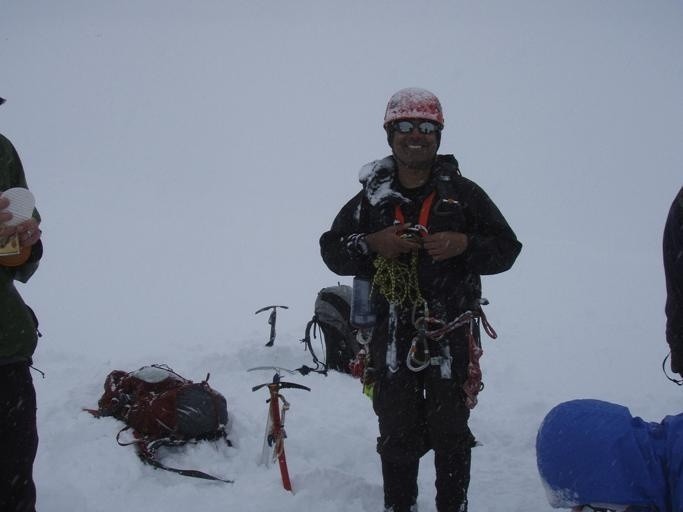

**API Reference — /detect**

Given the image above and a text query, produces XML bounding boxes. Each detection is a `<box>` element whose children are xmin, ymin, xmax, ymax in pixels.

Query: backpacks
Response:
<box><xmin>98</xmin><ymin>364</ymin><xmax>228</xmax><ymax>447</ymax></box>
<box><xmin>299</xmin><ymin>285</ymin><xmax>362</xmax><ymax>378</ymax></box>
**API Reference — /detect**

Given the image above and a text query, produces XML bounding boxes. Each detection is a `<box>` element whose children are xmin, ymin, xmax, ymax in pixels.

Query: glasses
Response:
<box><xmin>391</xmin><ymin>120</ymin><xmax>441</xmax><ymax>134</ymax></box>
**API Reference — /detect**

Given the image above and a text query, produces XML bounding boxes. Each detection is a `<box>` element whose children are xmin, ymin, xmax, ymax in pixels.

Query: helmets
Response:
<box><xmin>383</xmin><ymin>87</ymin><xmax>444</xmax><ymax>132</ymax></box>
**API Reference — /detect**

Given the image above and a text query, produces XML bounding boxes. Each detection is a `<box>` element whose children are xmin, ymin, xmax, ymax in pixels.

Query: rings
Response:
<box><xmin>27</xmin><ymin>230</ymin><xmax>33</xmax><ymax>235</ymax></box>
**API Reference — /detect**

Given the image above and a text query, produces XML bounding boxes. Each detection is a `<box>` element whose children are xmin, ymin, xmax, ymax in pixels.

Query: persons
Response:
<box><xmin>0</xmin><ymin>92</ymin><xmax>45</xmax><ymax>509</ymax></box>
<box><xmin>661</xmin><ymin>184</ymin><xmax>683</xmax><ymax>391</ymax></box>
<box><xmin>319</xmin><ymin>88</ymin><xmax>523</xmax><ymax>512</ymax></box>
<box><xmin>536</xmin><ymin>400</ymin><xmax>683</xmax><ymax>511</ymax></box>
